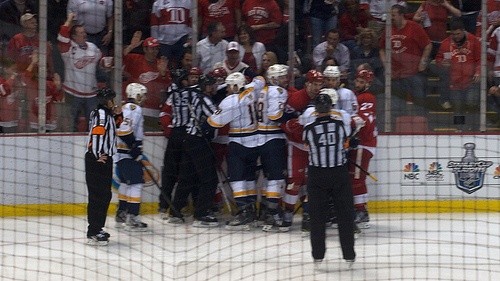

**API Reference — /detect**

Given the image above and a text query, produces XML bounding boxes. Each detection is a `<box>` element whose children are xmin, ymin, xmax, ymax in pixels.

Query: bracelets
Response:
<box><xmin>109</xmin><ymin>30</ymin><xmax>112</xmax><ymax>32</ymax></box>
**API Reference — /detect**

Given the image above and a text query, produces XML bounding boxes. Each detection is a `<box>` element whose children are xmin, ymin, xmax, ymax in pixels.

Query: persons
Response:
<box><xmin>115</xmin><ymin>83</ymin><xmax>149</xmax><ymax>233</ymax></box>
<box><xmin>85</xmin><ymin>89</ymin><xmax>122</xmax><ymax>246</ymax></box>
<box><xmin>0</xmin><ymin>0</ymin><xmax>500</xmax><ymax>132</ymax></box>
<box><xmin>302</xmin><ymin>95</ymin><xmax>355</xmax><ymax>264</ymax></box>
<box><xmin>159</xmin><ymin>65</ymin><xmax>376</xmax><ymax>233</ymax></box>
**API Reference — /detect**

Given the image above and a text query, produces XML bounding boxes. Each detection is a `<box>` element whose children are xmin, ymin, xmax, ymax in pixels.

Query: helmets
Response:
<box><xmin>125</xmin><ymin>83</ymin><xmax>147</xmax><ymax>103</ymax></box>
<box><xmin>319</xmin><ymin>87</ymin><xmax>338</xmax><ymax>107</ymax></box>
<box><xmin>188</xmin><ymin>67</ymin><xmax>203</xmax><ymax>76</ymax></box>
<box><xmin>225</xmin><ymin>72</ymin><xmax>246</xmax><ymax>93</ymax></box>
<box><xmin>324</xmin><ymin>66</ymin><xmax>341</xmax><ymax>88</ymax></box>
<box><xmin>210</xmin><ymin>67</ymin><xmax>226</xmax><ymax>79</ymax></box>
<box><xmin>305</xmin><ymin>69</ymin><xmax>323</xmax><ymax>92</ymax></box>
<box><xmin>95</xmin><ymin>87</ymin><xmax>117</xmax><ymax>107</ymax></box>
<box><xmin>356</xmin><ymin>69</ymin><xmax>374</xmax><ymax>92</ymax></box>
<box><xmin>265</xmin><ymin>63</ymin><xmax>288</xmax><ymax>86</ymax></box>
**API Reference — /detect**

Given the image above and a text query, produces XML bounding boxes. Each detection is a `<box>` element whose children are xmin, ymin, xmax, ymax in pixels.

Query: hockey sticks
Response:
<box><xmin>141</xmin><ymin>161</ymin><xmax>186</xmax><ymax>224</ymax></box>
<box><xmin>186</xmin><ymin>101</ymin><xmax>247</xmax><ymax>217</ymax></box>
<box><xmin>345</xmin><ymin>156</ymin><xmax>378</xmax><ymax>182</ymax></box>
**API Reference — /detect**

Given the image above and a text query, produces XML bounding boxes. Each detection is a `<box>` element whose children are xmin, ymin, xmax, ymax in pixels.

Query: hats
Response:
<box><xmin>142</xmin><ymin>37</ymin><xmax>161</xmax><ymax>49</ymax></box>
<box><xmin>226</xmin><ymin>41</ymin><xmax>240</xmax><ymax>51</ymax></box>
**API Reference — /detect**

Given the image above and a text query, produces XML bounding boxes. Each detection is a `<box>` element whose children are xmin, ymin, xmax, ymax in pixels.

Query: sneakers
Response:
<box><xmin>354</xmin><ymin>210</ymin><xmax>371</xmax><ymax>239</ymax></box>
<box><xmin>86</xmin><ymin>229</ymin><xmax>110</xmax><ymax>246</ymax></box>
<box><xmin>301</xmin><ymin>215</ymin><xmax>310</xmax><ymax>237</ymax></box>
<box><xmin>154</xmin><ymin>210</ymin><xmax>294</xmax><ymax>232</ymax></box>
<box><xmin>346</xmin><ymin>259</ymin><xmax>356</xmax><ymax>268</ymax></box>
<box><xmin>115</xmin><ymin>210</ymin><xmax>149</xmax><ymax>231</ymax></box>
<box><xmin>313</xmin><ymin>258</ymin><xmax>323</xmax><ymax>269</ymax></box>
